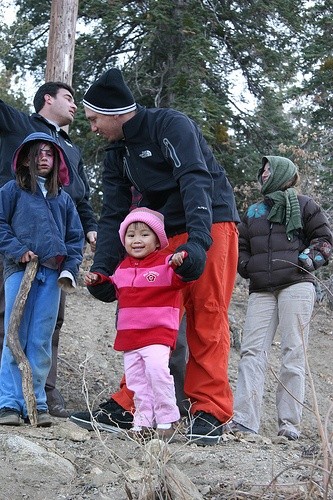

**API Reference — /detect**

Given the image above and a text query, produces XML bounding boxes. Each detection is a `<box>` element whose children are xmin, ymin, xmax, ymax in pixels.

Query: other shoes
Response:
<box><xmin>23</xmin><ymin>409</ymin><xmax>52</xmax><ymax>427</ymax></box>
<box><xmin>220</xmin><ymin>420</ymin><xmax>253</xmax><ymax>435</ymax></box>
<box><xmin>69</xmin><ymin>398</ymin><xmax>132</xmax><ymax>433</ymax></box>
<box><xmin>115</xmin><ymin>425</ymin><xmax>152</xmax><ymax>440</ymax></box>
<box><xmin>185</xmin><ymin>411</ymin><xmax>221</xmax><ymax>447</ymax></box>
<box><xmin>276</xmin><ymin>430</ymin><xmax>298</xmax><ymax>441</ymax></box>
<box><xmin>48</xmin><ymin>404</ymin><xmax>74</xmax><ymax>418</ymax></box>
<box><xmin>153</xmin><ymin>422</ymin><xmax>175</xmax><ymax>442</ymax></box>
<box><xmin>0</xmin><ymin>408</ymin><xmax>20</xmax><ymax>426</ymax></box>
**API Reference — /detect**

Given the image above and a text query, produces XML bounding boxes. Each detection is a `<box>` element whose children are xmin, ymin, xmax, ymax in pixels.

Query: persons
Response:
<box><xmin>222</xmin><ymin>157</ymin><xmax>333</xmax><ymax>439</ymax></box>
<box><xmin>0</xmin><ymin>132</ymin><xmax>83</xmax><ymax>426</ymax></box>
<box><xmin>0</xmin><ymin>81</ymin><xmax>97</xmax><ymax>417</ymax></box>
<box><xmin>84</xmin><ymin>207</ymin><xmax>188</xmax><ymax>442</ymax></box>
<box><xmin>70</xmin><ymin>69</ymin><xmax>237</xmax><ymax>443</ymax></box>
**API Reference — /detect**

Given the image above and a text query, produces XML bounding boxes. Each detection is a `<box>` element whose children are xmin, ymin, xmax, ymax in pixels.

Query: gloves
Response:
<box><xmin>86</xmin><ymin>262</ymin><xmax>115</xmax><ymax>303</ymax></box>
<box><xmin>170</xmin><ymin>230</ymin><xmax>213</xmax><ymax>282</ymax></box>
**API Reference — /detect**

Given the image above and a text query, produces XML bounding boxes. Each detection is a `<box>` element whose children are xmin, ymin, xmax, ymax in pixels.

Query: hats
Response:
<box><xmin>119</xmin><ymin>207</ymin><xmax>168</xmax><ymax>250</ymax></box>
<box><xmin>82</xmin><ymin>68</ymin><xmax>135</xmax><ymax>115</ymax></box>
